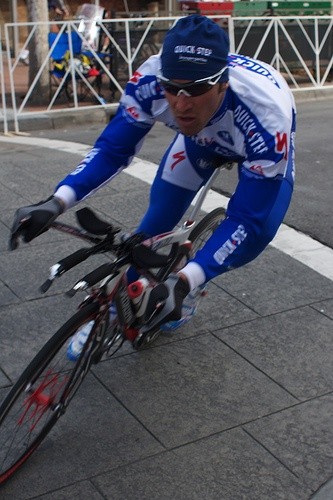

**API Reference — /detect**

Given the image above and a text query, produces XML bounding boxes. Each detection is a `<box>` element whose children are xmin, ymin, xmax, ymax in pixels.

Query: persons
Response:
<box><xmin>9</xmin><ymin>15</ymin><xmax>299</xmax><ymax>360</ymax></box>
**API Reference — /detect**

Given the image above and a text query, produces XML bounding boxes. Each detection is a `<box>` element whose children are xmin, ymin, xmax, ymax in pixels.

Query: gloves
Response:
<box><xmin>9</xmin><ymin>195</ymin><xmax>64</xmax><ymax>251</ymax></box>
<box><xmin>137</xmin><ymin>273</ymin><xmax>190</xmax><ymax>330</ymax></box>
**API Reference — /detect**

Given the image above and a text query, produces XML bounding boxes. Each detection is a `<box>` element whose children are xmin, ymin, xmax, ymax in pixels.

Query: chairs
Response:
<box><xmin>0</xmin><ymin>0</ymin><xmax>113</xmax><ymax>104</ymax></box>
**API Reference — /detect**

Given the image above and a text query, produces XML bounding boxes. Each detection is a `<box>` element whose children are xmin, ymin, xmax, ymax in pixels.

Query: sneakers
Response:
<box><xmin>67</xmin><ymin>320</ymin><xmax>95</xmax><ymax>361</ymax></box>
<box><xmin>160</xmin><ymin>283</ymin><xmax>207</xmax><ymax>333</ymax></box>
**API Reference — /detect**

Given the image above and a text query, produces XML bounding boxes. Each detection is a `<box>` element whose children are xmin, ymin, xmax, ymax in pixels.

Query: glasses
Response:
<box><xmin>158</xmin><ymin>64</ymin><xmax>227</xmax><ymax>97</ymax></box>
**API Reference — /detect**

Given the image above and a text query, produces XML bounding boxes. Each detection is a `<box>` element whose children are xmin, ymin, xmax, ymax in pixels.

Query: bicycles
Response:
<box><xmin>0</xmin><ymin>167</ymin><xmax>228</xmax><ymax>483</ymax></box>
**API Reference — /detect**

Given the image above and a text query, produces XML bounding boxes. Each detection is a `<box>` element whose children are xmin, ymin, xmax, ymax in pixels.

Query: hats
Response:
<box><xmin>161</xmin><ymin>15</ymin><xmax>230</xmax><ymax>82</ymax></box>
